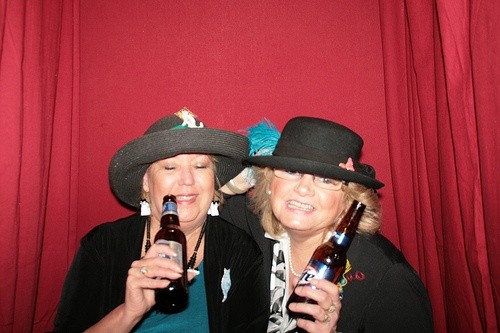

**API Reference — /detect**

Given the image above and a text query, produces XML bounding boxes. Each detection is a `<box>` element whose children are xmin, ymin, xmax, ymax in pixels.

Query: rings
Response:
<box><xmin>324</xmin><ymin>306</ymin><xmax>335</xmax><ymax>313</ymax></box>
<box><xmin>317</xmin><ymin>315</ymin><xmax>330</xmax><ymax>322</ymax></box>
<box><xmin>139</xmin><ymin>270</ymin><xmax>148</xmax><ymax>275</ymax></box>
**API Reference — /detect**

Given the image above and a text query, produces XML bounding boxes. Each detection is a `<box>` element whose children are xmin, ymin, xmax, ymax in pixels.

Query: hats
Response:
<box><xmin>108</xmin><ymin>111</ymin><xmax>251</xmax><ymax>212</ymax></box>
<box><xmin>246</xmin><ymin>116</ymin><xmax>385</xmax><ymax>191</ymax></box>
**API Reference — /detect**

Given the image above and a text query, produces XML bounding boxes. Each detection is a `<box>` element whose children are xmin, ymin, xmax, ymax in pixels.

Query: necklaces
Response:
<box><xmin>146</xmin><ymin>216</ymin><xmax>208</xmax><ymax>282</ymax></box>
<box><xmin>288</xmin><ymin>238</ymin><xmax>305</xmax><ymax>278</ymax></box>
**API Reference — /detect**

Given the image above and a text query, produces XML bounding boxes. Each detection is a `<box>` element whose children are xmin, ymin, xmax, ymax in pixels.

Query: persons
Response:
<box><xmin>54</xmin><ymin>111</ymin><xmax>274</xmax><ymax>333</ymax></box>
<box><xmin>241</xmin><ymin>117</ymin><xmax>437</xmax><ymax>333</ymax></box>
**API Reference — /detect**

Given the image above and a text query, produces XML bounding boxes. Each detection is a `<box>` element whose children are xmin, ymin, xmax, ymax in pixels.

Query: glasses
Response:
<box><xmin>273</xmin><ymin>166</ymin><xmax>348</xmax><ymax>191</ymax></box>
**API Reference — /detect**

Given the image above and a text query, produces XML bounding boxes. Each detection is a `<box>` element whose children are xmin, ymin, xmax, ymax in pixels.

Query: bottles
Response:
<box><xmin>154</xmin><ymin>194</ymin><xmax>189</xmax><ymax>315</ymax></box>
<box><xmin>286</xmin><ymin>200</ymin><xmax>366</xmax><ymax>322</ymax></box>
